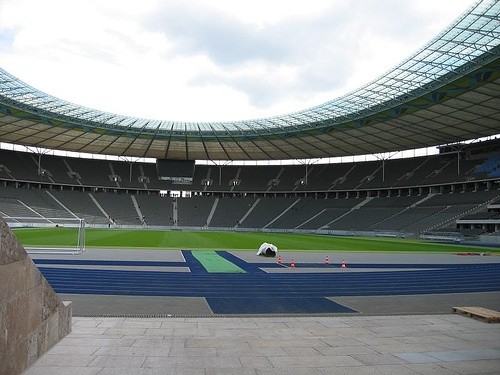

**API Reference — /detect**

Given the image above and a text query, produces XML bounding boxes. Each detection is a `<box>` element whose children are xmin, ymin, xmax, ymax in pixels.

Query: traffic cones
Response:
<box><xmin>340</xmin><ymin>259</ymin><xmax>347</xmax><ymax>269</ymax></box>
<box><xmin>278</xmin><ymin>255</ymin><xmax>283</xmax><ymax>264</ymax></box>
<box><xmin>325</xmin><ymin>256</ymin><xmax>331</xmax><ymax>265</ymax></box>
<box><xmin>288</xmin><ymin>259</ymin><xmax>296</xmax><ymax>268</ymax></box>
